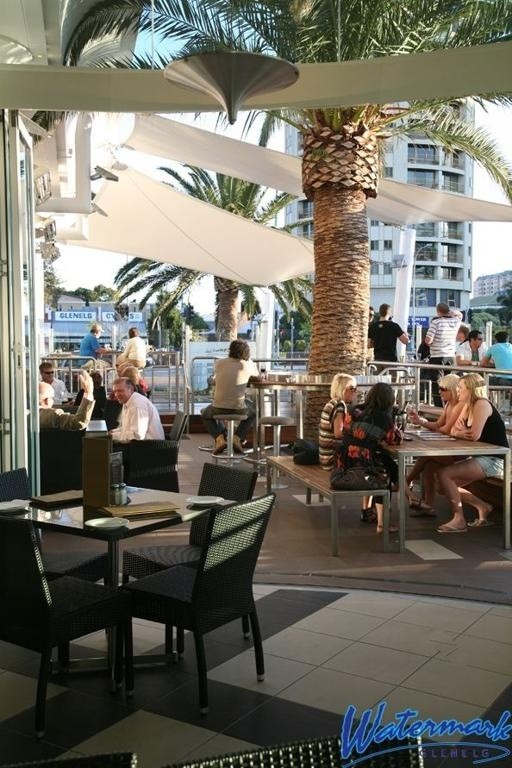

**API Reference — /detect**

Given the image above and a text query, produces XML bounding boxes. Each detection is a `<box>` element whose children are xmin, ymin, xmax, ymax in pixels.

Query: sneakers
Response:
<box><xmin>359</xmin><ymin>507</ymin><xmax>379</xmax><ymax>524</ymax></box>
<box><xmin>146</xmin><ymin>389</ymin><xmax>153</xmax><ymax>400</ymax></box>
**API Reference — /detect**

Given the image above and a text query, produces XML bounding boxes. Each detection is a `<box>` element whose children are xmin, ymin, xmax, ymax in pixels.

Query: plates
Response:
<box><xmin>186</xmin><ymin>503</ymin><xmax>223</xmax><ymax>510</ymax></box>
<box><xmin>186</xmin><ymin>496</ymin><xmax>224</xmax><ymax>505</ymax></box>
<box><xmin>83</xmin><ymin>517</ymin><xmax>131</xmax><ymax>530</ymax></box>
<box><xmin>1</xmin><ymin>499</ymin><xmax>30</xmax><ymax>513</ymax></box>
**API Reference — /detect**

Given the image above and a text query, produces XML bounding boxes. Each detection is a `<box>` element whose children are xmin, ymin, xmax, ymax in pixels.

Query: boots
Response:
<box><xmin>213</xmin><ymin>434</ymin><xmax>228</xmax><ymax>455</ymax></box>
<box><xmin>226</xmin><ymin>434</ymin><xmax>245</xmax><ymax>453</ymax></box>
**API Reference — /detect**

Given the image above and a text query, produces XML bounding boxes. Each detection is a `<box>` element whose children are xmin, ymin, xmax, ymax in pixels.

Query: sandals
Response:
<box><xmin>408</xmin><ymin>497</ymin><xmax>439</xmax><ymax>517</ymax></box>
<box><xmin>376</xmin><ymin>523</ymin><xmax>400</xmax><ymax>534</ymax></box>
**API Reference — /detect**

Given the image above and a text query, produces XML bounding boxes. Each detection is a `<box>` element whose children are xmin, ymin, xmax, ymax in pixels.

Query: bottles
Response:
<box><xmin>110</xmin><ymin>483</ymin><xmax>128</xmax><ymax>506</ymax></box>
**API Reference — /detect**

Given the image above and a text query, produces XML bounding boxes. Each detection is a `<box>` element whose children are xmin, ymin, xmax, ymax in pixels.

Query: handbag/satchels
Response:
<box><xmin>285</xmin><ymin>438</ymin><xmax>318</xmax><ymax>465</ymax></box>
<box><xmin>329</xmin><ymin>463</ymin><xmax>387</xmax><ymax>490</ymax></box>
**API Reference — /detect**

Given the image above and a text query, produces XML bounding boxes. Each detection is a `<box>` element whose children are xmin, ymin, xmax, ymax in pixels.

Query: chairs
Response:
<box><xmin>1</xmin><ymin>352</ymin><xmax>512</xmax><ymax>767</ymax></box>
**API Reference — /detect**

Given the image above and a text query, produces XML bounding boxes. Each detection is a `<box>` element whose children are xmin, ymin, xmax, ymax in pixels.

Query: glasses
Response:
<box><xmin>347</xmin><ymin>388</ymin><xmax>357</xmax><ymax>392</ymax></box>
<box><xmin>42</xmin><ymin>371</ymin><xmax>54</xmax><ymax>374</ymax></box>
<box><xmin>439</xmin><ymin>387</ymin><xmax>447</xmax><ymax>391</ymax></box>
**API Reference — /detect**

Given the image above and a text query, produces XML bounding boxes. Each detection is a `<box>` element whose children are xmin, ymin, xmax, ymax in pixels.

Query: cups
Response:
<box><xmin>399</xmin><ymin>352</ymin><xmax>421</xmax><ymax>362</ymax></box>
<box><xmin>354</xmin><ymin>375</ymin><xmax>391</xmax><ymax>384</ymax></box>
<box><xmin>395</xmin><ymin>414</ymin><xmax>407</xmax><ymax>436</ymax></box>
<box><xmin>290</xmin><ymin>371</ymin><xmax>327</xmax><ymax>383</ymax></box>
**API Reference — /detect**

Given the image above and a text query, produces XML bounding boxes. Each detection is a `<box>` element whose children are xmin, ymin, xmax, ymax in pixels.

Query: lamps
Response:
<box><xmin>89</xmin><ymin>164</ymin><xmax>119</xmax><ymax>183</ymax></box>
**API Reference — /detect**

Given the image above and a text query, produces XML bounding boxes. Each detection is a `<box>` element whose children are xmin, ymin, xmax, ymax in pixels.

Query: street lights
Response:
<box><xmin>412</xmin><ymin>241</ymin><xmax>435</xmax><ymax>360</ymax></box>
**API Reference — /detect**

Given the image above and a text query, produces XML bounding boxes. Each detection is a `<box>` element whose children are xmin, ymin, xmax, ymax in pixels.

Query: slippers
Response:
<box><xmin>434</xmin><ymin>524</ymin><xmax>468</xmax><ymax>533</ymax></box>
<box><xmin>467</xmin><ymin>518</ymin><xmax>496</xmax><ymax>527</ymax></box>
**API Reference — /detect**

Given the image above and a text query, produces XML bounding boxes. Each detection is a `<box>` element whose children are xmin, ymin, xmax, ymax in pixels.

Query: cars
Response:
<box><xmin>404</xmin><ymin>351</ymin><xmax>429</xmax><ymax>365</ymax></box>
<box><xmin>60</xmin><ymin>350</ymin><xmax>85</xmax><ymax>383</ymax></box>
<box><xmin>146</xmin><ymin>354</ymin><xmax>155</xmax><ymax>369</ymax></box>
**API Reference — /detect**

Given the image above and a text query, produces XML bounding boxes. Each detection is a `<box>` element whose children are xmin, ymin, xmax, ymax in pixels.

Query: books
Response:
<box><xmin>102</xmin><ymin>499</ymin><xmax>180</xmax><ymax>517</ymax></box>
<box><xmin>28</xmin><ymin>488</ymin><xmax>84</xmax><ymax>510</ymax></box>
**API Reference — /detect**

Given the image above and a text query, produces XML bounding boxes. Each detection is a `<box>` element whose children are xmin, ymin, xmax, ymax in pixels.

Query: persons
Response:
<box><xmin>110</xmin><ymin>376</ymin><xmax>166</xmax><ymax>443</ymax></box>
<box><xmin>368</xmin><ymin>302</ymin><xmax>512</xmax><ymax>407</ymax></box>
<box><xmin>35</xmin><ymin>322</ymin><xmax>148</xmax><ymax>430</ymax></box>
<box><xmin>436</xmin><ymin>371</ymin><xmax>509</xmax><ymax>532</ymax></box>
<box><xmin>334</xmin><ymin>382</ymin><xmax>437</xmax><ymax>532</ymax></box>
<box><xmin>201</xmin><ymin>340</ymin><xmax>260</xmax><ymax>454</ymax></box>
<box><xmin>317</xmin><ymin>373</ymin><xmax>386</xmax><ymax>524</ymax></box>
<box><xmin>405</xmin><ymin>372</ymin><xmax>470</xmax><ymax>518</ymax></box>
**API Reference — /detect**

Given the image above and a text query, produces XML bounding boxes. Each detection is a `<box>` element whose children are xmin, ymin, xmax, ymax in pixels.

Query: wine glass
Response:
<box><xmin>260</xmin><ymin>364</ymin><xmax>266</xmax><ymax>382</ymax></box>
<box><xmin>406</xmin><ymin>404</ymin><xmax>416</xmax><ymax>428</ymax></box>
